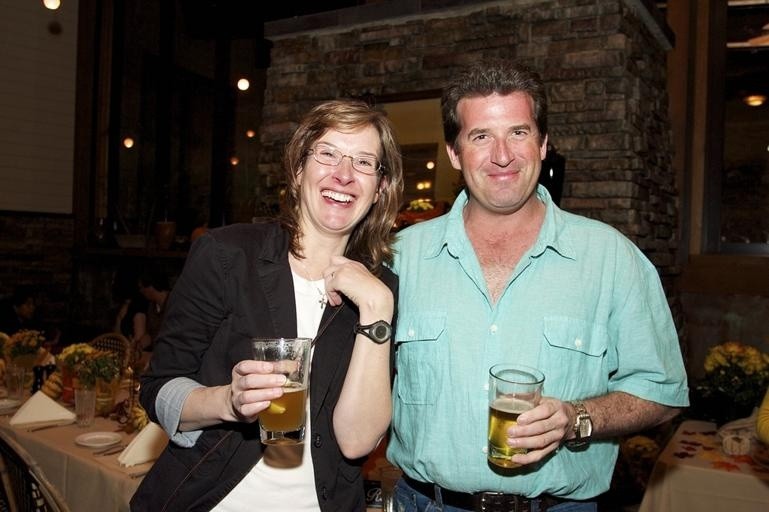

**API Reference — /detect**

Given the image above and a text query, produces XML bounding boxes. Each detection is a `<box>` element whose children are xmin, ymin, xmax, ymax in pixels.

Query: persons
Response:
<box><xmin>0</xmin><ymin>284</ymin><xmax>54</xmax><ymax>343</ymax></box>
<box><xmin>379</xmin><ymin>54</ymin><xmax>696</xmax><ymax>511</ymax></box>
<box><xmin>101</xmin><ymin>269</ymin><xmax>147</xmax><ymax>346</ymax></box>
<box><xmin>137</xmin><ymin>265</ymin><xmax>171</xmax><ymax>353</ymax></box>
<box><xmin>127</xmin><ymin>96</ymin><xmax>401</xmax><ymax>511</ymax></box>
<box><xmin>756</xmin><ymin>385</ymin><xmax>769</xmax><ymax>449</ymax></box>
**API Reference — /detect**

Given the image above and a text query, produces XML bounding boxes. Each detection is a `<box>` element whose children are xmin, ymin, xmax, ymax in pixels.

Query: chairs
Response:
<box><xmin>1</xmin><ymin>431</ymin><xmax>69</xmax><ymax>512</ymax></box>
<box><xmin>86</xmin><ymin>331</ymin><xmax>134</xmax><ymax>416</ymax></box>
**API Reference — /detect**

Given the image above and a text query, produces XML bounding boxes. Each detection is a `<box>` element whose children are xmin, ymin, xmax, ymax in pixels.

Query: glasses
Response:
<box><xmin>305</xmin><ymin>142</ymin><xmax>388</xmax><ymax>175</ymax></box>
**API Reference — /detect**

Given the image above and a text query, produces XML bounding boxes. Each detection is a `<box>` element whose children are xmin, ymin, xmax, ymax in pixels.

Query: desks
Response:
<box><xmin>635</xmin><ymin>418</ymin><xmax>768</xmax><ymax>511</ymax></box>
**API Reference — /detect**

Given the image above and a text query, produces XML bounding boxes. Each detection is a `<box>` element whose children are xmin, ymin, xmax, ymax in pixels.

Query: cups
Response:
<box><xmin>250</xmin><ymin>336</ymin><xmax>311</xmax><ymax>444</ymax></box>
<box><xmin>484</xmin><ymin>363</ymin><xmax>546</xmax><ymax>468</ymax></box>
<box><xmin>6</xmin><ymin>366</ymin><xmax>25</xmax><ymax>403</ymax></box>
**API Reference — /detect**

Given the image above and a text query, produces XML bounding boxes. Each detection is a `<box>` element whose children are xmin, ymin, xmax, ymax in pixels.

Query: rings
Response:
<box><xmin>331</xmin><ymin>271</ymin><xmax>335</xmax><ymax>278</ymax></box>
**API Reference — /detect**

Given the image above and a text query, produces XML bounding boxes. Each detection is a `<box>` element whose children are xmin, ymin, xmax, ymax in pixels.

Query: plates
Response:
<box><xmin>0</xmin><ymin>398</ymin><xmax>22</xmax><ymax>410</ymax></box>
<box><xmin>75</xmin><ymin>431</ymin><xmax>122</xmax><ymax>447</ymax></box>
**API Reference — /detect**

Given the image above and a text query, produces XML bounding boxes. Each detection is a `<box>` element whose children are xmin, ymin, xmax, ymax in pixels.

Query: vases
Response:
<box><xmin>5</xmin><ymin>358</ymin><xmax>27</xmax><ymax>401</ymax></box>
<box><xmin>714</xmin><ymin>397</ymin><xmax>754</xmax><ymax>429</ymax></box>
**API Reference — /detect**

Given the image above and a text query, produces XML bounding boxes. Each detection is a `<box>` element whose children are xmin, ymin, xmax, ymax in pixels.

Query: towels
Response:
<box><xmin>10</xmin><ymin>387</ymin><xmax>80</xmax><ymax>430</ymax></box>
<box><xmin>118</xmin><ymin>419</ymin><xmax>170</xmax><ymax>466</ymax></box>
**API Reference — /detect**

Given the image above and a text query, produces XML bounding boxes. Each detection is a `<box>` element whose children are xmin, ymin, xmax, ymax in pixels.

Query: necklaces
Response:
<box><xmin>297</xmin><ymin>247</ymin><xmax>329</xmax><ymax>311</ymax></box>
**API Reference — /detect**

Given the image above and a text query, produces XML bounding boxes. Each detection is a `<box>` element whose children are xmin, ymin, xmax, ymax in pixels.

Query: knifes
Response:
<box><xmin>30</xmin><ymin>421</ymin><xmax>76</xmax><ymax>431</ymax></box>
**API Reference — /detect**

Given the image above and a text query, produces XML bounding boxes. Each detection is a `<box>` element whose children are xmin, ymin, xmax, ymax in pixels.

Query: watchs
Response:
<box><xmin>566</xmin><ymin>400</ymin><xmax>595</xmax><ymax>453</ymax></box>
<box><xmin>352</xmin><ymin>320</ymin><xmax>395</xmax><ymax>344</ymax></box>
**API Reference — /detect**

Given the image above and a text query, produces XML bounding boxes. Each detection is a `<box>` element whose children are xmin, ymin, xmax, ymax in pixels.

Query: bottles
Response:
<box><xmin>31</xmin><ymin>363</ymin><xmax>59</xmax><ymax>394</ymax></box>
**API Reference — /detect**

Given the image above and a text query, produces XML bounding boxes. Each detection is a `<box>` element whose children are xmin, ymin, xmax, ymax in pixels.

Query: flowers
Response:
<box><xmin>3</xmin><ymin>326</ymin><xmax>49</xmax><ymax>362</ymax></box>
<box><xmin>623</xmin><ymin>435</ymin><xmax>660</xmax><ymax>461</ymax></box>
<box><xmin>59</xmin><ymin>342</ymin><xmax>122</xmax><ymax>387</ymax></box>
<box><xmin>701</xmin><ymin>337</ymin><xmax>769</xmax><ymax>400</ymax></box>
